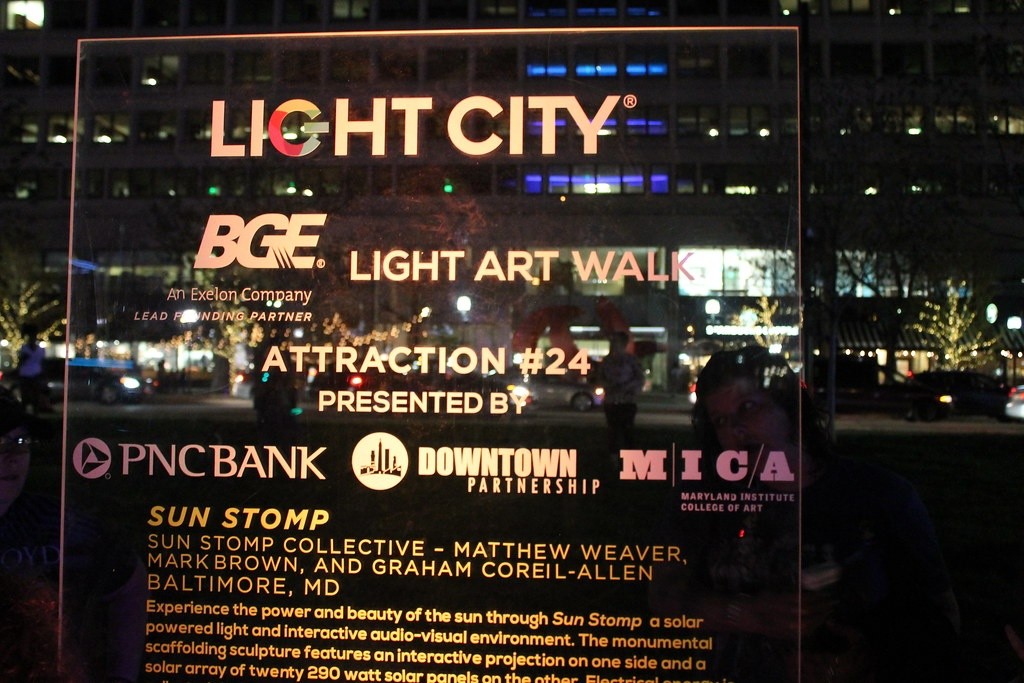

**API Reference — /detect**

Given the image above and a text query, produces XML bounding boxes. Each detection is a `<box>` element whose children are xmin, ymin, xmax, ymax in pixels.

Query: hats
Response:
<box><xmin>0</xmin><ymin>384</ymin><xmax>39</xmax><ymax>434</ymax></box>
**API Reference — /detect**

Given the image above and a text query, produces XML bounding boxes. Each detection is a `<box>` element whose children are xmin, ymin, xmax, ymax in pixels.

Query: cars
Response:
<box><xmin>14</xmin><ymin>313</ymin><xmax>891</xmax><ymax>419</ymax></box>
<box><xmin>1003</xmin><ymin>384</ymin><xmax>1024</xmax><ymax>422</ymax></box>
<box><xmin>884</xmin><ymin>368</ymin><xmax>1004</xmax><ymax>425</ymax></box>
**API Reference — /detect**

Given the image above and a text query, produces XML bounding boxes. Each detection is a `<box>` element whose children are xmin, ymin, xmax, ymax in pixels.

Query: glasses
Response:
<box><xmin>0</xmin><ymin>433</ymin><xmax>31</xmax><ymax>454</ymax></box>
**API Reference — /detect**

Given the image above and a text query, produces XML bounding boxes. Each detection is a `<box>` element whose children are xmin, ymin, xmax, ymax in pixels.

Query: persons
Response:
<box><xmin>18</xmin><ymin>338</ymin><xmax>47</xmax><ymax>412</ymax></box>
<box><xmin>586</xmin><ymin>330</ymin><xmax>648</xmax><ymax>453</ymax></box>
<box><xmin>251</xmin><ymin>337</ymin><xmax>297</xmax><ymax>456</ymax></box>
<box><xmin>650</xmin><ymin>347</ymin><xmax>962</xmax><ymax>683</ymax></box>
<box><xmin>0</xmin><ymin>384</ymin><xmax>146</xmax><ymax>682</ymax></box>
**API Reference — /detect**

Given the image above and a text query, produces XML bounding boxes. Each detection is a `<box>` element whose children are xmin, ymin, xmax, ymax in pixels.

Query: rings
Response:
<box><xmin>828</xmin><ymin>665</ymin><xmax>835</xmax><ymax>676</ymax></box>
<box><xmin>833</xmin><ymin>654</ymin><xmax>838</xmax><ymax>665</ymax></box>
